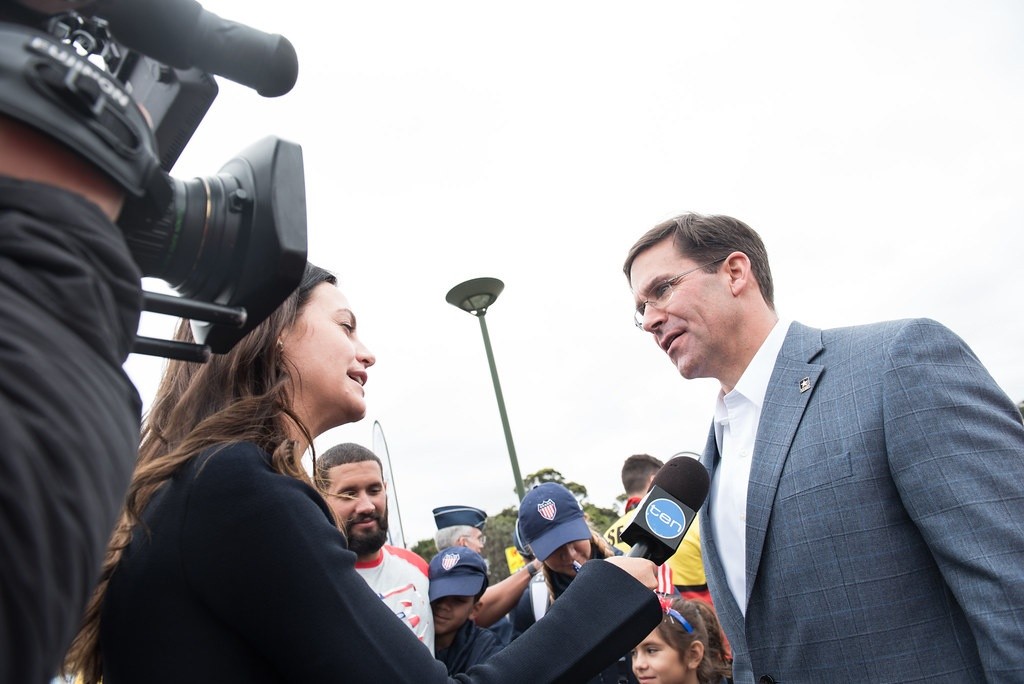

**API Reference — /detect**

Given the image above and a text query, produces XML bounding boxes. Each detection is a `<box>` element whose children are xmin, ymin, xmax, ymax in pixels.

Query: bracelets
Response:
<box><xmin>528</xmin><ymin>563</ymin><xmax>537</xmax><ymax>577</ymax></box>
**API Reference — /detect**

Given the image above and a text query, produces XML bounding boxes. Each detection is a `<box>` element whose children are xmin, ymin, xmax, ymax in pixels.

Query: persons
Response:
<box><xmin>433</xmin><ymin>505</ymin><xmax>543</xmax><ymax>647</ymax></box>
<box><xmin>631</xmin><ymin>598</ymin><xmax>732</xmax><ymax>684</ymax></box>
<box><xmin>511</xmin><ymin>483</ymin><xmax>682</xmax><ymax>648</ymax></box>
<box><xmin>624</xmin><ymin>213</ymin><xmax>1024</xmax><ymax>684</ymax></box>
<box><xmin>315</xmin><ymin>443</ymin><xmax>434</xmax><ymax>660</ymax></box>
<box><xmin>514</xmin><ymin>515</ymin><xmax>624</xmax><ymax>634</ymax></box>
<box><xmin>0</xmin><ymin>121</ymin><xmax>143</xmax><ymax>684</ymax></box>
<box><xmin>608</xmin><ymin>455</ymin><xmax>713</xmax><ymax>609</ymax></box>
<box><xmin>69</xmin><ymin>261</ymin><xmax>657</xmax><ymax>684</ymax></box>
<box><xmin>428</xmin><ymin>546</ymin><xmax>505</xmax><ymax>676</ymax></box>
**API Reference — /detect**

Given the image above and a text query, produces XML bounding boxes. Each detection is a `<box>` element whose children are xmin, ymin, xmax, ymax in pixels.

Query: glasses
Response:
<box><xmin>632</xmin><ymin>257</ymin><xmax>727</xmax><ymax>331</ymax></box>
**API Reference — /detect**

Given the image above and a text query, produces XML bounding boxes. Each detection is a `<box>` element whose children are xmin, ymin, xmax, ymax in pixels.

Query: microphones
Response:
<box><xmin>621</xmin><ymin>457</ymin><xmax>711</xmax><ymax>566</ymax></box>
<box><xmin>73</xmin><ymin>0</ymin><xmax>298</xmax><ymax>98</ymax></box>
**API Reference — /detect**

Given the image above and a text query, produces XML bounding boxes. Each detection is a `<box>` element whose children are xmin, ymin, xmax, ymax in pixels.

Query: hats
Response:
<box><xmin>433</xmin><ymin>505</ymin><xmax>487</xmax><ymax>531</ymax></box>
<box><xmin>520</xmin><ymin>482</ymin><xmax>592</xmax><ymax>561</ymax></box>
<box><xmin>513</xmin><ymin>518</ymin><xmax>531</xmax><ymax>556</ymax></box>
<box><xmin>428</xmin><ymin>546</ymin><xmax>487</xmax><ymax>601</ymax></box>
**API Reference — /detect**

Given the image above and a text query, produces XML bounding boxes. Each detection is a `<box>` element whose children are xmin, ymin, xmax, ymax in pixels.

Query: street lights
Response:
<box><xmin>443</xmin><ymin>276</ymin><xmax>526</xmax><ymax>502</ymax></box>
